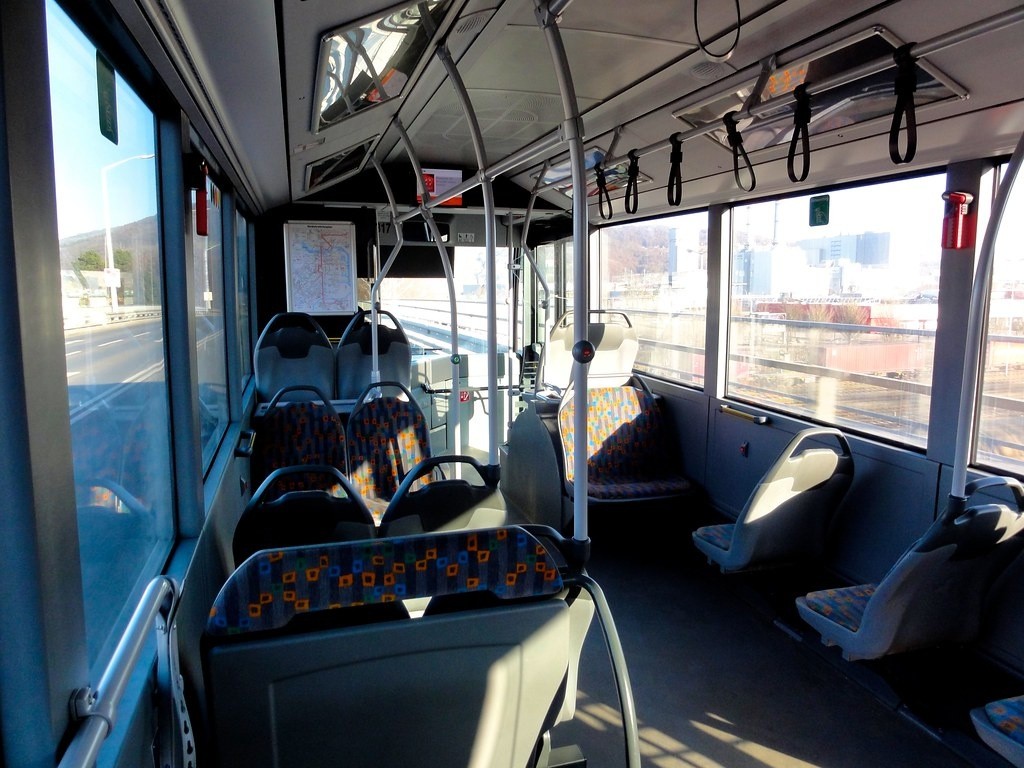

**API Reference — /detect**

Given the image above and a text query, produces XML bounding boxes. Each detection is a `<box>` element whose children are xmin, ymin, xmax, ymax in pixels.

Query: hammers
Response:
<box><xmin>941</xmin><ymin>192</ymin><xmax>973</xmax><ymax>214</ymax></box>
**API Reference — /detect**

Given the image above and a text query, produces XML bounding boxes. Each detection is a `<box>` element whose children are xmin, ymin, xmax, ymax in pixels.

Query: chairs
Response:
<box><xmin>197</xmin><ymin>310</ymin><xmax>599</xmax><ymax>768</ymax></box>
<box><xmin>691</xmin><ymin>427</ymin><xmax>856</xmax><ymax>572</ymax></box>
<box><xmin>558</xmin><ymin>374</ymin><xmax>689</xmax><ymax>528</ymax></box>
<box><xmin>794</xmin><ymin>475</ymin><xmax>1024</xmax><ymax>662</ymax></box>
<box><xmin>521</xmin><ymin>310</ymin><xmax>639</xmax><ymax>405</ymax></box>
<box><xmin>968</xmin><ymin>692</ymin><xmax>1024</xmax><ymax>768</ymax></box>
